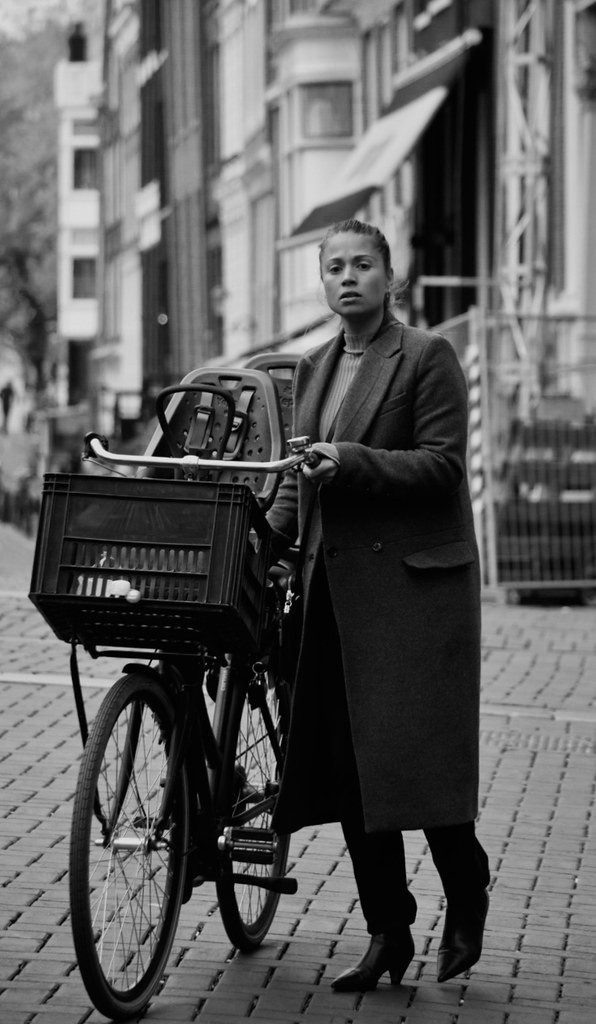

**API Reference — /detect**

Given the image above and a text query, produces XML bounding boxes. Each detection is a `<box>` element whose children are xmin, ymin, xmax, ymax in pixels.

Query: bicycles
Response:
<box><xmin>29</xmin><ymin>434</ymin><xmax>320</xmax><ymax>1024</ymax></box>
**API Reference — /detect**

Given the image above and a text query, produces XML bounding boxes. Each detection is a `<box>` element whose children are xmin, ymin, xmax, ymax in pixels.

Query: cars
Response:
<box><xmin>0</xmin><ymin>389</ymin><xmax>87</xmax><ymax>537</ymax></box>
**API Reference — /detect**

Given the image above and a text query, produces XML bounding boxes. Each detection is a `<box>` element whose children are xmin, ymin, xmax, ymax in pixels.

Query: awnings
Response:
<box><xmin>273</xmin><ymin>30</ymin><xmax>492</xmax><ymax>255</ymax></box>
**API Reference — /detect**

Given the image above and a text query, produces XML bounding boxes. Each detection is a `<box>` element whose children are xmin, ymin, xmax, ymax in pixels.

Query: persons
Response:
<box><xmin>67</xmin><ymin>19</ymin><xmax>88</xmax><ymax>62</ymax></box>
<box><xmin>0</xmin><ymin>381</ymin><xmax>15</xmax><ymax>429</ymax></box>
<box><xmin>245</xmin><ymin>220</ymin><xmax>491</xmax><ymax>991</ymax></box>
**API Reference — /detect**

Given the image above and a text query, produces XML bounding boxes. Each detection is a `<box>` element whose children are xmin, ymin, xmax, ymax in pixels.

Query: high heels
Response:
<box><xmin>330</xmin><ymin>918</ymin><xmax>416</xmax><ymax>993</ymax></box>
<box><xmin>437</xmin><ymin>890</ymin><xmax>490</xmax><ymax>983</ymax></box>
